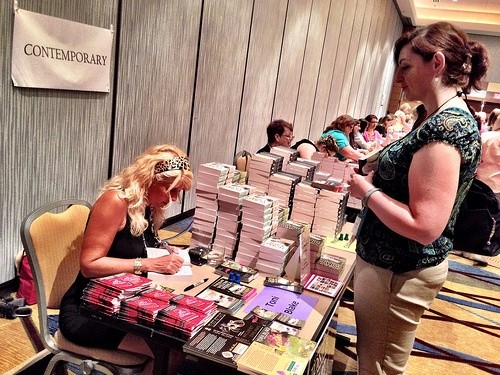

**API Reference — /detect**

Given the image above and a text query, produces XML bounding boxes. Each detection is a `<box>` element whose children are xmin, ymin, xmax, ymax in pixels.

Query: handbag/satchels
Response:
<box><xmin>453</xmin><ymin>105</ymin><xmax>500</xmax><ymax>257</ymax></box>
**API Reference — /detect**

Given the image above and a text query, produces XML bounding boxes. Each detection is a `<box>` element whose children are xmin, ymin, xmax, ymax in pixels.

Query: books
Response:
<box><xmin>81</xmin><ymin>248</ymin><xmax>318</xmax><ymax>375</ymax></box>
<box><xmin>190</xmin><ymin>145</ymin><xmax>365</xmax><ymax>298</ymax></box>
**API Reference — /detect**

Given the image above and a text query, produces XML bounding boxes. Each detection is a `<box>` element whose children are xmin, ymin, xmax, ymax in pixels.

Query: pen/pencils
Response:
<box><xmin>163</xmin><ymin>242</ymin><xmax>173</xmax><ymax>254</ymax></box>
<box><xmin>183</xmin><ymin>277</ymin><xmax>208</xmax><ymax>292</ymax></box>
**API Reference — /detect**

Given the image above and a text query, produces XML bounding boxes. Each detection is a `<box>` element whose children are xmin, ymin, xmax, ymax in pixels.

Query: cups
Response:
<box><xmin>207</xmin><ymin>245</ymin><xmax>225</xmax><ymax>267</ymax></box>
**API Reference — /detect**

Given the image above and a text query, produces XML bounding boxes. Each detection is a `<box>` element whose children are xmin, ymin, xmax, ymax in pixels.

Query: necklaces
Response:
<box><xmin>142</xmin><ymin>204</ymin><xmax>161</xmax><ymax>249</ymax></box>
<box><xmin>378</xmin><ymin>91</ymin><xmax>464</xmax><ymax>152</ymax></box>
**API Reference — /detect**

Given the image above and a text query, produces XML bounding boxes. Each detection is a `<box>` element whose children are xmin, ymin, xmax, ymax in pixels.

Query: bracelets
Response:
<box><xmin>133</xmin><ymin>258</ymin><xmax>142</xmax><ymax>273</ymax></box>
<box><xmin>362</xmin><ymin>188</ymin><xmax>382</xmax><ymax>208</ymax></box>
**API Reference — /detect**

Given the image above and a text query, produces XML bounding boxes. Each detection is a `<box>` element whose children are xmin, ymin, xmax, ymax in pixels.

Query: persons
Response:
<box><xmin>346</xmin><ymin>21</ymin><xmax>491</xmax><ymax>375</ymax></box>
<box><xmin>59</xmin><ymin>144</ymin><xmax>194</xmax><ymax>375</ymax></box>
<box><xmin>290</xmin><ymin>135</ymin><xmax>339</xmax><ymax>162</ymax></box>
<box><xmin>256</xmin><ymin>119</ymin><xmax>294</xmax><ymax>153</ymax></box>
<box><xmin>453</xmin><ymin>105</ymin><xmax>500</xmax><ymax>257</ymax></box>
<box><xmin>321</xmin><ymin>103</ymin><xmax>419</xmax><ymax>160</ymax></box>
